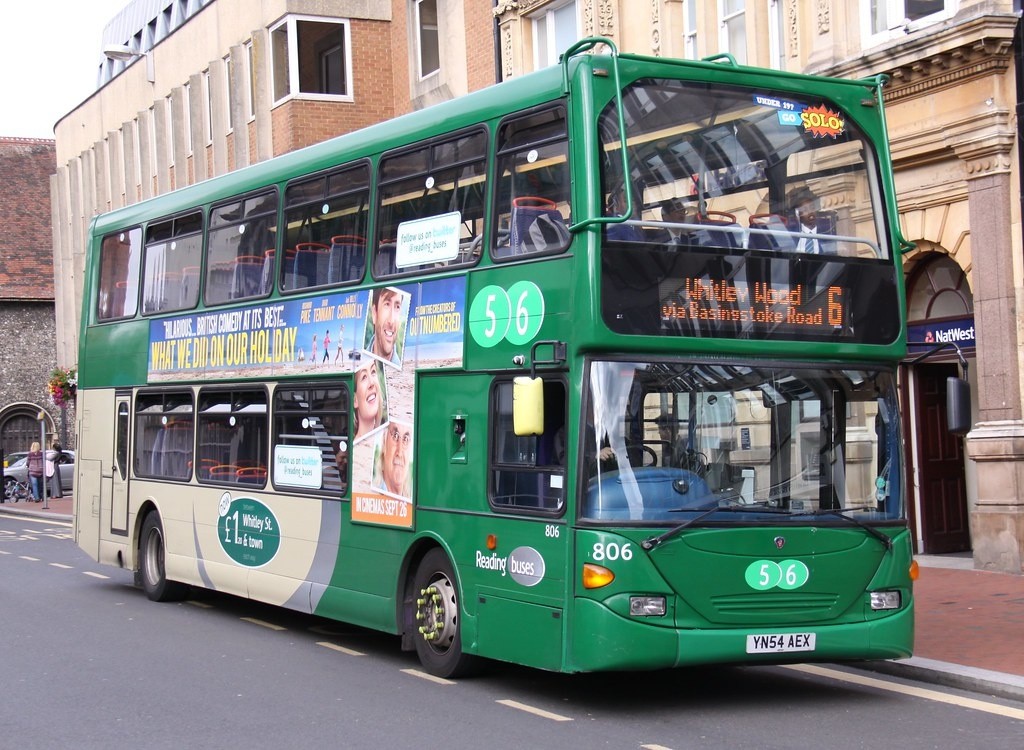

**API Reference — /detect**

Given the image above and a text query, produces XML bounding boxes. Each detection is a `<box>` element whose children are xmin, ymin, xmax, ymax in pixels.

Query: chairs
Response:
<box><xmin>150</xmin><ymin>420</ymin><xmax>267</xmax><ymax>486</ymax></box>
<box><xmin>605</xmin><ymin>211</ymin><xmax>797</xmax><ymax>253</ymax></box>
<box><xmin>104</xmin><ymin>196</ymin><xmax>569</xmax><ymax>318</ymax></box>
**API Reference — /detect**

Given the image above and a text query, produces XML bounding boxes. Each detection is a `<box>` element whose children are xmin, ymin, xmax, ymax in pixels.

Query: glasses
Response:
<box><xmin>389</xmin><ymin>429</ymin><xmax>411</xmax><ymax>446</ymax></box>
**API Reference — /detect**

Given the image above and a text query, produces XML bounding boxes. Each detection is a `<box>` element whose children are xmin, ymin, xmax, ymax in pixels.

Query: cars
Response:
<box><xmin>3</xmin><ymin>448</ymin><xmax>75</xmax><ymax>499</ymax></box>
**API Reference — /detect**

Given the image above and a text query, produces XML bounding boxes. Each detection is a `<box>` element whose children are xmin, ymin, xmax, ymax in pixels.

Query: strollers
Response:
<box><xmin>4</xmin><ymin>468</ymin><xmax>35</xmax><ymax>503</ymax></box>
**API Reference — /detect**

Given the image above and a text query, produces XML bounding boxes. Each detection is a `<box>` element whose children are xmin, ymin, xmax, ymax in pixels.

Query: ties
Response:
<box><xmin>805</xmin><ymin>232</ymin><xmax>814</xmax><ymax>253</ymax></box>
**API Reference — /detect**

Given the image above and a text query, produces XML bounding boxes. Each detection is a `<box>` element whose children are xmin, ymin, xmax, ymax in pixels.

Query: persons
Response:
<box><xmin>309</xmin><ymin>334</ymin><xmax>318</xmax><ymax>366</ymax></box>
<box><xmin>773</xmin><ymin>198</ymin><xmax>835</xmax><ymax>254</ymax></box>
<box><xmin>555</xmin><ymin>404</ymin><xmax>636</xmax><ymax>478</ymax></box>
<box><xmin>651</xmin><ymin>198</ymin><xmax>690</xmax><ymax>245</ymax></box>
<box><xmin>353</xmin><ymin>360</ymin><xmax>386</xmax><ymax>440</ymax></box>
<box><xmin>46</xmin><ymin>443</ymin><xmax>62</xmax><ymax>499</ymax></box>
<box><xmin>365</xmin><ymin>287</ymin><xmax>404</xmax><ymax>365</ymax></box>
<box><xmin>59</xmin><ymin>455</ymin><xmax>68</xmax><ymax>464</ymax></box>
<box><xmin>655</xmin><ymin>413</ymin><xmax>686</xmax><ymax>468</ymax></box>
<box><xmin>334</xmin><ymin>324</ymin><xmax>345</xmax><ymax>364</ymax></box>
<box><xmin>27</xmin><ymin>442</ymin><xmax>43</xmax><ymax>502</ymax></box>
<box><xmin>322</xmin><ymin>330</ymin><xmax>331</xmax><ymax>364</ymax></box>
<box><xmin>373</xmin><ymin>423</ymin><xmax>411</xmax><ymax>501</ymax></box>
<box><xmin>606</xmin><ymin>189</ymin><xmax>647</xmax><ymax>242</ymax></box>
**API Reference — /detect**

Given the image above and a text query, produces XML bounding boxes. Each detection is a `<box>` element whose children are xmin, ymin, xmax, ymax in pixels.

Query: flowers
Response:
<box><xmin>48</xmin><ymin>366</ymin><xmax>78</xmax><ymax>409</ymax></box>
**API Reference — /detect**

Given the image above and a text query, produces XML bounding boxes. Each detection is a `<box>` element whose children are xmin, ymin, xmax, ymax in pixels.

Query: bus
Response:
<box><xmin>70</xmin><ymin>34</ymin><xmax>974</xmax><ymax>680</ymax></box>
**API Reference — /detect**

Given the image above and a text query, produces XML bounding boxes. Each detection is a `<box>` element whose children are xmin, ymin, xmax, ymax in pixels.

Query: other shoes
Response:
<box><xmin>35</xmin><ymin>499</ymin><xmax>40</xmax><ymax>503</ymax></box>
